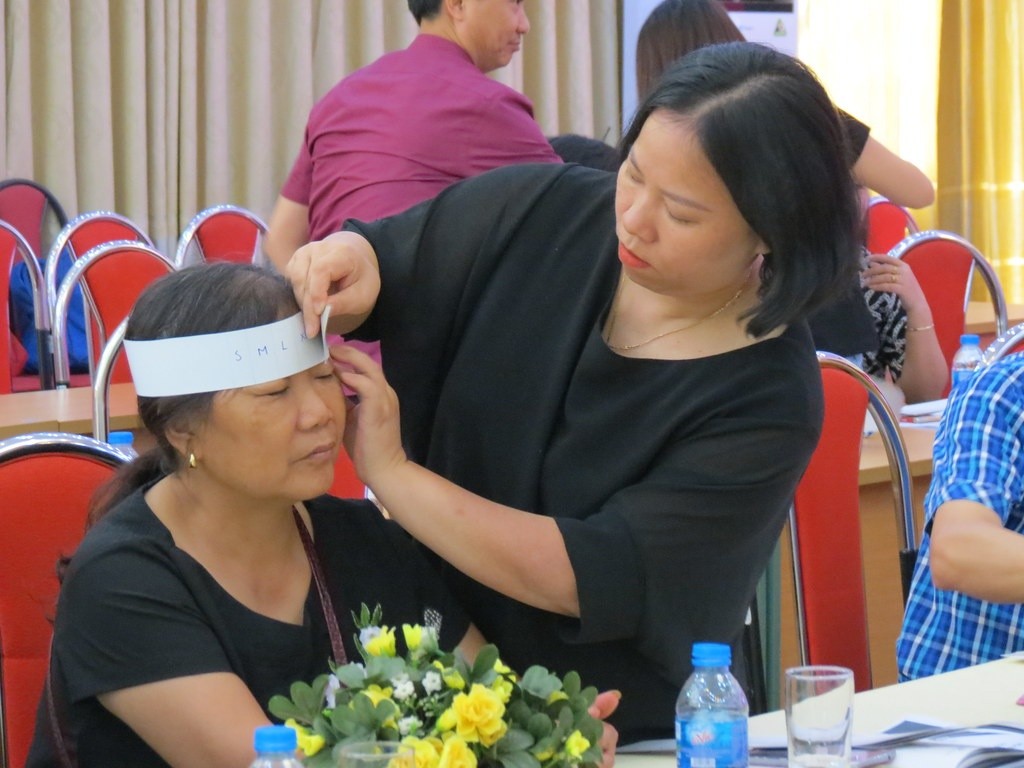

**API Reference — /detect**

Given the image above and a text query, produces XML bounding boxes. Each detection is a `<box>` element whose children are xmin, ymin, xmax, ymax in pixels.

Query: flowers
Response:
<box><xmin>267</xmin><ymin>604</ymin><xmax>603</xmax><ymax>768</ymax></box>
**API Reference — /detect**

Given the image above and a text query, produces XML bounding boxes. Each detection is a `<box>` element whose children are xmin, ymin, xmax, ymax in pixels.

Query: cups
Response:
<box><xmin>782</xmin><ymin>663</ymin><xmax>854</xmax><ymax>768</ymax></box>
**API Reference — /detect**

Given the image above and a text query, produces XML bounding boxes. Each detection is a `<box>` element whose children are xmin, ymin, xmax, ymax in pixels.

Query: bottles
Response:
<box><xmin>668</xmin><ymin>642</ymin><xmax>752</xmax><ymax>768</ymax></box>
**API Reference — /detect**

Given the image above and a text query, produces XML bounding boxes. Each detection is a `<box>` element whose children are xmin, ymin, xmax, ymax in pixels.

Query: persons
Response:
<box><xmin>27</xmin><ymin>262</ymin><xmax>625</xmax><ymax>768</ymax></box>
<box><xmin>283</xmin><ymin>45</ymin><xmax>856</xmax><ymax>752</ymax></box>
<box><xmin>856</xmin><ymin>244</ymin><xmax>949</xmax><ymax>421</ymax></box>
<box><xmin>637</xmin><ymin>0</ymin><xmax>935</xmax><ymax>368</ymax></box>
<box><xmin>898</xmin><ymin>353</ymin><xmax>1024</xmax><ymax>684</ymax></box>
<box><xmin>263</xmin><ymin>0</ymin><xmax>567</xmax><ymax>405</ymax></box>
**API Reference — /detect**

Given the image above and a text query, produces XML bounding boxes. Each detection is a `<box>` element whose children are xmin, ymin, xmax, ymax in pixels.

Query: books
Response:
<box><xmin>746</xmin><ymin>713</ymin><xmax>1024</xmax><ymax>768</ymax></box>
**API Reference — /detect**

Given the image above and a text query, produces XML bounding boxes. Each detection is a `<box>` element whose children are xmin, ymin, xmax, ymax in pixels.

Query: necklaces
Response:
<box><xmin>604</xmin><ymin>260</ymin><xmax>756</xmax><ymax>350</ymax></box>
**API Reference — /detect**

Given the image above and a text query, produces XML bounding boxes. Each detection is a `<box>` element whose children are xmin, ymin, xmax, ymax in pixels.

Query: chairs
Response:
<box><xmin>973</xmin><ymin>322</ymin><xmax>1024</xmax><ymax>374</ymax></box>
<box><xmin>95</xmin><ymin>309</ymin><xmax>372</xmax><ymax>501</ymax></box>
<box><xmin>0</xmin><ymin>221</ymin><xmax>54</xmax><ymax>396</ymax></box>
<box><xmin>56</xmin><ymin>240</ymin><xmax>180</xmax><ymax>394</ymax></box>
<box><xmin>853</xmin><ymin>196</ymin><xmax>920</xmax><ymax>255</ymax></box>
<box><xmin>0</xmin><ymin>432</ymin><xmax>151</xmax><ymax>768</ymax></box>
<box><xmin>882</xmin><ymin>228</ymin><xmax>1008</xmax><ymax>406</ymax></box>
<box><xmin>175</xmin><ymin>205</ymin><xmax>273</xmax><ymax>273</ymax></box>
<box><xmin>756</xmin><ymin>349</ymin><xmax>923</xmax><ymax>715</ymax></box>
<box><xmin>1</xmin><ymin>177</ymin><xmax>90</xmax><ymax>393</ymax></box>
<box><xmin>44</xmin><ymin>209</ymin><xmax>154</xmax><ymax>384</ymax></box>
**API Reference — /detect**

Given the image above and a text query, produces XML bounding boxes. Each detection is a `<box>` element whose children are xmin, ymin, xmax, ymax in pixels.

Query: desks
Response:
<box><xmin>610</xmin><ymin>652</ymin><xmax>1024</xmax><ymax>768</ymax></box>
<box><xmin>962</xmin><ymin>298</ymin><xmax>1024</xmax><ymax>354</ymax></box>
<box><xmin>0</xmin><ymin>389</ymin><xmax>158</xmax><ymax>460</ymax></box>
<box><xmin>757</xmin><ymin>399</ymin><xmax>961</xmax><ymax>709</ymax></box>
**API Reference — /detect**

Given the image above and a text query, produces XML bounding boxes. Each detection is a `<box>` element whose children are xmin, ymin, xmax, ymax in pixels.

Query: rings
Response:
<box><xmin>893</xmin><ymin>266</ymin><xmax>898</xmax><ymax>274</ymax></box>
<box><xmin>891</xmin><ymin>274</ymin><xmax>896</xmax><ymax>283</ymax></box>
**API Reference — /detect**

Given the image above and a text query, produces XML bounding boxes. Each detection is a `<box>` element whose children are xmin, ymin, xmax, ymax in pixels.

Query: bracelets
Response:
<box><xmin>904</xmin><ymin>323</ymin><xmax>936</xmax><ymax>333</ymax></box>
